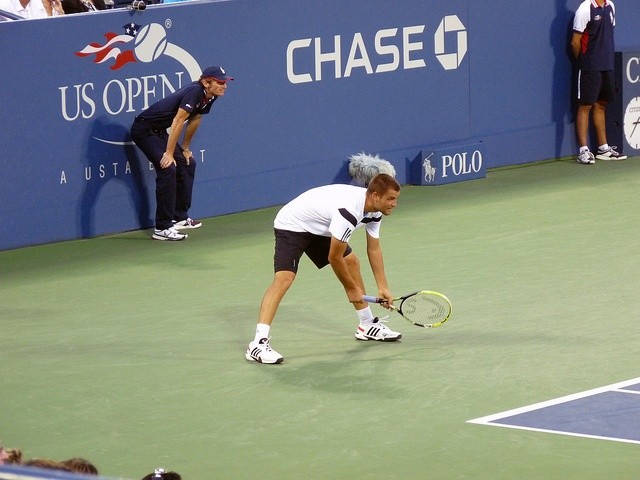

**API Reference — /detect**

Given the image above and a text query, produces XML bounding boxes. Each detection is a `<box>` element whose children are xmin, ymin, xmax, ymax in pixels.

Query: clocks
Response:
<box><xmin>623</xmin><ymin>96</ymin><xmax>640</xmax><ymax>151</ymax></box>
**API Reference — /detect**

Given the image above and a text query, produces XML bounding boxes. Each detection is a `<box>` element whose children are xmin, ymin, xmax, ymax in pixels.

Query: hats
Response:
<box><xmin>203</xmin><ymin>66</ymin><xmax>235</xmax><ymax>82</ymax></box>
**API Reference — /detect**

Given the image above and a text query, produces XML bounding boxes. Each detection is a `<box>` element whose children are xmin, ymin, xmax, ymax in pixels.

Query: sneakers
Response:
<box><xmin>151</xmin><ymin>228</ymin><xmax>187</xmax><ymax>241</ymax></box>
<box><xmin>576</xmin><ymin>148</ymin><xmax>595</xmax><ymax>164</ymax></box>
<box><xmin>172</xmin><ymin>219</ymin><xmax>202</xmax><ymax>229</ymax></box>
<box><xmin>356</xmin><ymin>317</ymin><xmax>402</xmax><ymax>341</ymax></box>
<box><xmin>245</xmin><ymin>341</ymin><xmax>283</xmax><ymax>364</ymax></box>
<box><xmin>596</xmin><ymin>146</ymin><xmax>627</xmax><ymax>161</ymax></box>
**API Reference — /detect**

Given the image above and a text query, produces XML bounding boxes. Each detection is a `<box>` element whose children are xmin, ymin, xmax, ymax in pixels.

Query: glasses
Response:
<box><xmin>151</xmin><ymin>467</ymin><xmax>165</xmax><ymax>479</ymax></box>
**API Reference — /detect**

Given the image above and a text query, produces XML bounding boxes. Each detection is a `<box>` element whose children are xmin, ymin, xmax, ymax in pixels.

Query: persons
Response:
<box><xmin>128</xmin><ymin>63</ymin><xmax>235</xmax><ymax>243</ymax></box>
<box><xmin>27</xmin><ymin>0</ymin><xmax>65</xmax><ymax>18</ymax></box>
<box><xmin>569</xmin><ymin>0</ymin><xmax>629</xmax><ymax>164</ymax></box>
<box><xmin>245</xmin><ymin>170</ymin><xmax>405</xmax><ymax>366</ymax></box>
<box><xmin>2</xmin><ymin>0</ymin><xmax>30</xmax><ymax>20</ymax></box>
